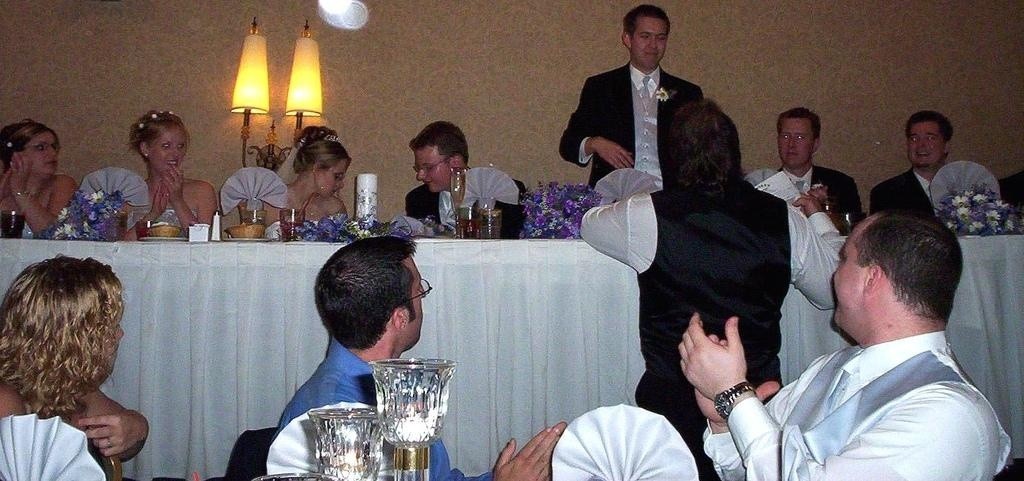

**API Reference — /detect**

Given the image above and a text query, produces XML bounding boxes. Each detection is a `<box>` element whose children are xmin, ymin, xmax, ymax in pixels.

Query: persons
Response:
<box><xmin>234</xmin><ymin>125</ymin><xmax>352</xmax><ymax>230</ymax></box>
<box><xmin>0</xmin><ymin>255</ymin><xmax>149</xmax><ymax>481</ymax></box>
<box><xmin>679</xmin><ymin>211</ymin><xmax>1009</xmax><ymax>481</ymax></box>
<box><xmin>270</xmin><ymin>235</ymin><xmax>568</xmax><ymax>481</ymax></box>
<box><xmin>127</xmin><ymin>110</ymin><xmax>218</xmax><ymax>238</ymax></box>
<box><xmin>745</xmin><ymin>107</ymin><xmax>861</xmax><ymax>236</ymax></box>
<box><xmin>869</xmin><ymin>110</ymin><xmax>954</xmax><ymax>226</ymax></box>
<box><xmin>403</xmin><ymin>121</ymin><xmax>527</xmax><ymax>239</ymax></box>
<box><xmin>583</xmin><ymin>97</ymin><xmax>848</xmax><ymax>481</ymax></box>
<box><xmin>0</xmin><ymin>119</ymin><xmax>79</xmax><ymax>240</ymax></box>
<box><xmin>560</xmin><ymin>4</ymin><xmax>704</xmax><ymax>191</ymax></box>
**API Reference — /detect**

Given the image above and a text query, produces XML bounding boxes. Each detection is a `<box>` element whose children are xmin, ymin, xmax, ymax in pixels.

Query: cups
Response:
<box><xmin>307</xmin><ymin>405</ymin><xmax>384</xmax><ymax>480</ymax></box>
<box><xmin>0</xmin><ymin>209</ymin><xmax>24</xmax><ymax>238</ymax></box>
<box><xmin>458</xmin><ymin>207</ymin><xmax>481</xmax><ymax>239</ymax></box>
<box><xmin>480</xmin><ymin>210</ymin><xmax>503</xmax><ymax>239</ymax></box>
<box><xmin>278</xmin><ymin>207</ymin><xmax>306</xmax><ymax>240</ymax></box>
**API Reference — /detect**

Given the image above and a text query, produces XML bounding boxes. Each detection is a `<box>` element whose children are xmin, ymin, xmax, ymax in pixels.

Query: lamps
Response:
<box><xmin>230</xmin><ymin>15</ymin><xmax>326</xmax><ymax>168</ymax></box>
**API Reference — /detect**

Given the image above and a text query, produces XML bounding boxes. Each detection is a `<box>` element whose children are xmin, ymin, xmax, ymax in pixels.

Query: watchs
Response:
<box><xmin>714</xmin><ymin>382</ymin><xmax>755</xmax><ymax>418</ymax></box>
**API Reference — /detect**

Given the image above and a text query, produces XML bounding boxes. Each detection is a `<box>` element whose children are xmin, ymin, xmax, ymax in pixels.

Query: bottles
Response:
<box><xmin>355</xmin><ymin>173</ymin><xmax>377</xmax><ymax>226</ymax></box>
<box><xmin>209</xmin><ymin>210</ymin><xmax>221</xmax><ymax>243</ymax></box>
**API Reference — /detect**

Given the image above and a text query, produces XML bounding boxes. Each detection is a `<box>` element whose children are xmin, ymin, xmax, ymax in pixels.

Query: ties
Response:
<box><xmin>795</xmin><ymin>179</ymin><xmax>807</xmax><ymax>196</ymax></box>
<box><xmin>641</xmin><ymin>75</ymin><xmax>654</xmax><ymax>111</ymax></box>
<box><xmin>806</xmin><ymin>351</ymin><xmax>864</xmax><ymax>431</ymax></box>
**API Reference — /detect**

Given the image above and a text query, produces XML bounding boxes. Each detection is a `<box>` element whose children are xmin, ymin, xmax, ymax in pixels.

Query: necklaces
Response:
<box><xmin>286</xmin><ymin>193</ymin><xmax>314</xmax><ymax>219</ymax></box>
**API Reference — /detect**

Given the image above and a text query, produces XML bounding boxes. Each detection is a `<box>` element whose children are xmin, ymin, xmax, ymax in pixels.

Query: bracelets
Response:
<box><xmin>14</xmin><ymin>189</ymin><xmax>33</xmax><ymax>204</ymax></box>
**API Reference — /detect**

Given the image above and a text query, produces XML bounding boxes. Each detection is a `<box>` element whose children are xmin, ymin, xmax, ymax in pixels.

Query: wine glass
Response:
<box><xmin>448</xmin><ymin>166</ymin><xmax>465</xmax><ymax>238</ymax></box>
<box><xmin>374</xmin><ymin>357</ymin><xmax>457</xmax><ymax>479</ymax></box>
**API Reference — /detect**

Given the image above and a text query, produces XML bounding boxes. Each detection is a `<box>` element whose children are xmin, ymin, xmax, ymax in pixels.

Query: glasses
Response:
<box><xmin>404</xmin><ymin>276</ymin><xmax>436</xmax><ymax>304</ymax></box>
<box><xmin>412</xmin><ymin>155</ymin><xmax>452</xmax><ymax>174</ymax></box>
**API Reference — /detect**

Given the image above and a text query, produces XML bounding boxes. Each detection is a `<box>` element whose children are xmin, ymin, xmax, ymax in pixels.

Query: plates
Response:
<box><xmin>222</xmin><ymin>238</ymin><xmax>273</xmax><ymax>242</ymax></box>
<box><xmin>140</xmin><ymin>236</ymin><xmax>187</xmax><ymax>243</ymax></box>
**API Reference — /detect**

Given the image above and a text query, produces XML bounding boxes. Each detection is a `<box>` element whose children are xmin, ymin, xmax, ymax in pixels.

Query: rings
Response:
<box><xmin>107</xmin><ymin>437</ymin><xmax>112</xmax><ymax>448</ymax></box>
<box><xmin>168</xmin><ymin>181</ymin><xmax>175</xmax><ymax>185</ymax></box>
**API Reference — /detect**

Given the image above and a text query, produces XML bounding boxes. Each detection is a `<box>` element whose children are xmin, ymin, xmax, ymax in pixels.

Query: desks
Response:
<box><xmin>0</xmin><ymin>232</ymin><xmax>1022</xmax><ymax>481</ymax></box>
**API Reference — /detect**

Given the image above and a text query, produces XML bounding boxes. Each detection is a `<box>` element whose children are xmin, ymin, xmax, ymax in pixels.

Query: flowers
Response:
<box><xmin>931</xmin><ymin>185</ymin><xmax>1023</xmax><ymax>236</ymax></box>
<box><xmin>513</xmin><ymin>177</ymin><xmax>601</xmax><ymax>240</ymax></box>
<box><xmin>36</xmin><ymin>185</ymin><xmax>127</xmax><ymax>240</ymax></box>
<box><xmin>294</xmin><ymin>208</ymin><xmax>410</xmax><ymax>244</ymax></box>
